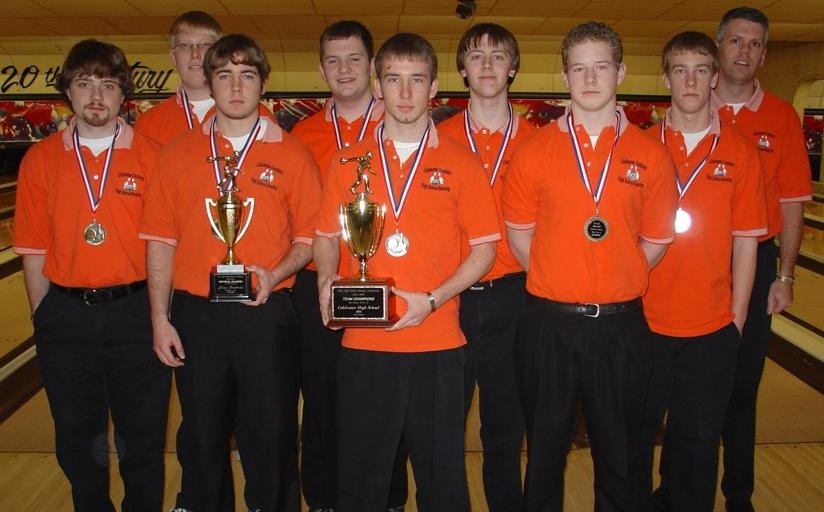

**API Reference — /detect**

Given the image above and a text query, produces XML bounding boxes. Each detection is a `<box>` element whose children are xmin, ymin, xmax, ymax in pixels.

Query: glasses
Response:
<box><xmin>172</xmin><ymin>41</ymin><xmax>213</xmax><ymax>51</ymax></box>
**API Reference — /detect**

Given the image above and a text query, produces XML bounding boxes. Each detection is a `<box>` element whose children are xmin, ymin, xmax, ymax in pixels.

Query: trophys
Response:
<box><xmin>194</xmin><ymin>152</ymin><xmax>257</xmax><ymax>303</ymax></box>
<box><xmin>326</xmin><ymin>151</ymin><xmax>401</xmax><ymax>328</ymax></box>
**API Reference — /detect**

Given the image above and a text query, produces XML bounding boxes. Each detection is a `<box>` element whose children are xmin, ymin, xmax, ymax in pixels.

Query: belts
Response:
<box><xmin>549</xmin><ymin>301</ymin><xmax>629</xmax><ymax>318</ymax></box>
<box><xmin>469</xmin><ymin>280</ymin><xmax>493</xmax><ymax>294</ymax></box>
<box><xmin>54</xmin><ymin>282</ymin><xmax>145</xmax><ymax>307</ymax></box>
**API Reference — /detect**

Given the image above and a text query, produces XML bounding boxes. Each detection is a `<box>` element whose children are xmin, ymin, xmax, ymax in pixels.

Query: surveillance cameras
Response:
<box><xmin>455</xmin><ymin>0</ymin><xmax>477</xmax><ymax>19</ymax></box>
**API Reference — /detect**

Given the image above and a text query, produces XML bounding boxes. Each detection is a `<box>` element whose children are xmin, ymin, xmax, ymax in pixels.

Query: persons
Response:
<box><xmin>13</xmin><ymin>38</ymin><xmax>173</xmax><ymax>512</ymax></box>
<box><xmin>502</xmin><ymin>21</ymin><xmax>674</xmax><ymax>512</ymax></box>
<box><xmin>642</xmin><ymin>29</ymin><xmax>768</xmax><ymax>512</ymax></box>
<box><xmin>319</xmin><ymin>32</ymin><xmax>501</xmax><ymax>511</ymax></box>
<box><xmin>652</xmin><ymin>5</ymin><xmax>813</xmax><ymax>511</ymax></box>
<box><xmin>129</xmin><ymin>10</ymin><xmax>273</xmax><ymax>464</ymax></box>
<box><xmin>137</xmin><ymin>33</ymin><xmax>321</xmax><ymax>512</ymax></box>
<box><xmin>288</xmin><ymin>21</ymin><xmax>408</xmax><ymax>510</ymax></box>
<box><xmin>417</xmin><ymin>22</ymin><xmax>539</xmax><ymax>509</ymax></box>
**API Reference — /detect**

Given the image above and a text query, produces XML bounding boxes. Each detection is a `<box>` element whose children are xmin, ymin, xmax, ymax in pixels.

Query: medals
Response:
<box><xmin>584</xmin><ymin>212</ymin><xmax>609</xmax><ymax>242</ymax></box>
<box><xmin>381</xmin><ymin>231</ymin><xmax>409</xmax><ymax>257</ymax></box>
<box><xmin>669</xmin><ymin>206</ymin><xmax>693</xmax><ymax>235</ymax></box>
<box><xmin>81</xmin><ymin>220</ymin><xmax>104</xmax><ymax>245</ymax></box>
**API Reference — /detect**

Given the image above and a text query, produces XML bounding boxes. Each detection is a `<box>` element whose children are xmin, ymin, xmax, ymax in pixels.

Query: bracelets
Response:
<box><xmin>774</xmin><ymin>271</ymin><xmax>797</xmax><ymax>287</ymax></box>
<box><xmin>423</xmin><ymin>288</ymin><xmax>437</xmax><ymax>315</ymax></box>
